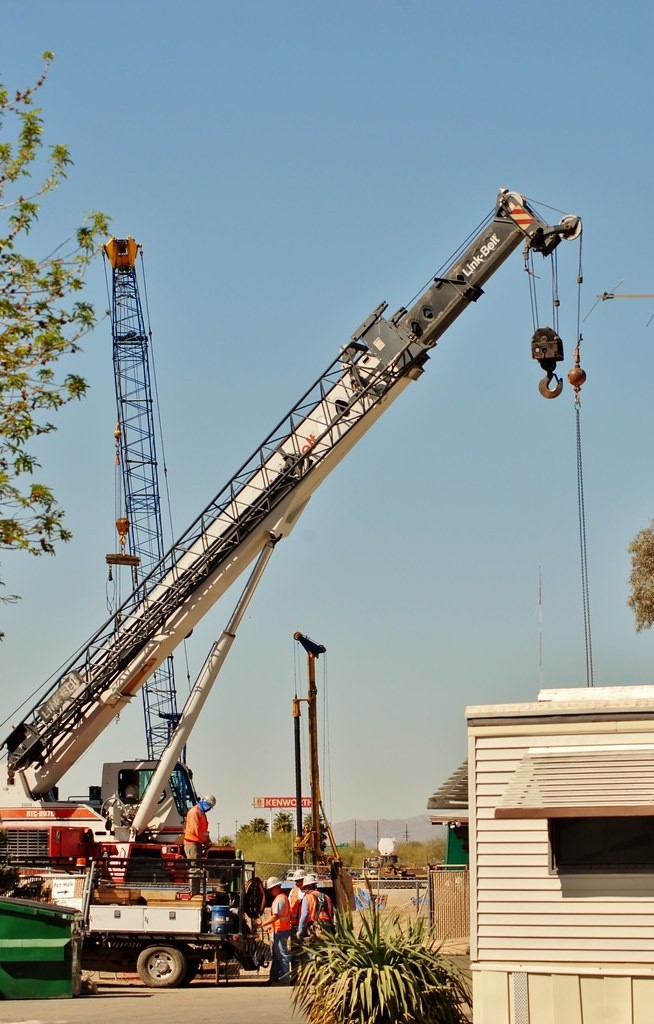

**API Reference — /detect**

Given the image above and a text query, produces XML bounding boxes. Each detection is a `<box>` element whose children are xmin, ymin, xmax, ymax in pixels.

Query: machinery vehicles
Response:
<box><xmin>0</xmin><ymin>184</ymin><xmax>582</xmax><ymax>892</ymax></box>
<box><xmin>0</xmin><ymin>856</ymin><xmax>247</xmax><ymax>989</ymax></box>
<box><xmin>290</xmin><ymin>631</ymin><xmax>426</xmax><ymax>891</ymax></box>
<box><xmin>74</xmin><ymin>232</ymin><xmax>209</xmax><ymax>844</ymax></box>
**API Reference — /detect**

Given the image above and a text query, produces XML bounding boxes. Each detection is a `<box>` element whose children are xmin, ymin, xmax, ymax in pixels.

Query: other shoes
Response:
<box><xmin>266</xmin><ymin>972</ymin><xmax>299</xmax><ymax>987</ymax></box>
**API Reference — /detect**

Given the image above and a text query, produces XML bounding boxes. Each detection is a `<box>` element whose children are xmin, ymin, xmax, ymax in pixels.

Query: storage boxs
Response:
<box><xmin>91</xmin><ymin>888</ymin><xmax>141</xmax><ymax>907</ymax></box>
<box><xmin>146</xmin><ymin>899</ymin><xmax>202</xmax><ymax>907</ymax></box>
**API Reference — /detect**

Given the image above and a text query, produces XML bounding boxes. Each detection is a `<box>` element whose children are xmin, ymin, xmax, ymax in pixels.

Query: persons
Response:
<box><xmin>296</xmin><ymin>876</ymin><xmax>334</xmax><ymax>959</ymax></box>
<box><xmin>288</xmin><ymin>869</ymin><xmax>307</xmax><ymax>985</ymax></box>
<box><xmin>256</xmin><ymin>876</ymin><xmax>291</xmax><ymax>985</ymax></box>
<box><xmin>183</xmin><ymin>795</ymin><xmax>216</xmax><ymax>900</ymax></box>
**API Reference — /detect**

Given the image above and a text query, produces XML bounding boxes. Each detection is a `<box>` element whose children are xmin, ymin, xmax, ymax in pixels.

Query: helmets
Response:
<box><xmin>267</xmin><ymin>876</ymin><xmax>282</xmax><ymax>890</ymax></box>
<box><xmin>291</xmin><ymin>870</ymin><xmax>308</xmax><ymax>881</ymax></box>
<box><xmin>302</xmin><ymin>875</ymin><xmax>319</xmax><ymax>887</ymax></box>
<box><xmin>203</xmin><ymin>795</ymin><xmax>217</xmax><ymax>809</ymax></box>
<box><xmin>129</xmin><ymin>773</ymin><xmax>138</xmax><ymax>778</ymax></box>
